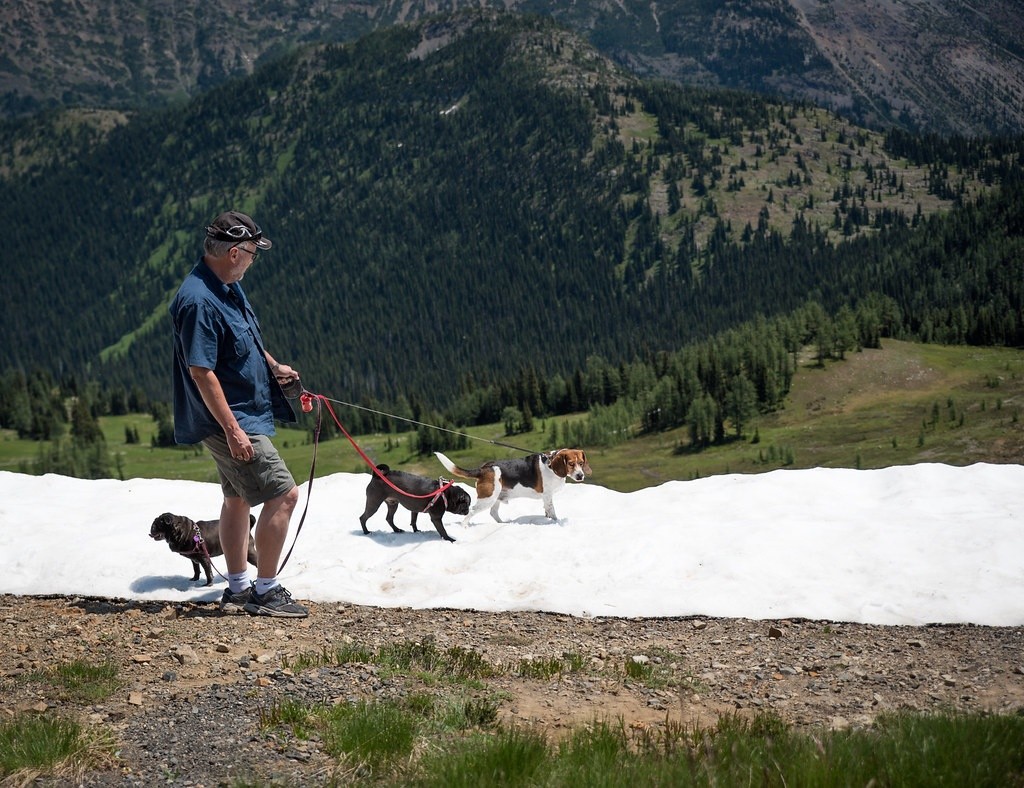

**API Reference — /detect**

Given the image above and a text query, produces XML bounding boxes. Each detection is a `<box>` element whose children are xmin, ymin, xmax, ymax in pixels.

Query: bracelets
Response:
<box><xmin>273</xmin><ymin>362</ymin><xmax>280</xmax><ymax>371</ymax></box>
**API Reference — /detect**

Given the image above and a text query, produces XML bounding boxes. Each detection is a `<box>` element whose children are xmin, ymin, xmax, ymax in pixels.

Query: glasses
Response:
<box><xmin>227</xmin><ymin>222</ymin><xmax>262</xmax><ymax>252</ymax></box>
<box><xmin>228</xmin><ymin>246</ymin><xmax>258</xmax><ymax>261</ymax></box>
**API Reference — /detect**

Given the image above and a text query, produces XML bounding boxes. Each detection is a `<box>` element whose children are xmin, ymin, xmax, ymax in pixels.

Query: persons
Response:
<box><xmin>170</xmin><ymin>211</ymin><xmax>309</xmax><ymax>617</ymax></box>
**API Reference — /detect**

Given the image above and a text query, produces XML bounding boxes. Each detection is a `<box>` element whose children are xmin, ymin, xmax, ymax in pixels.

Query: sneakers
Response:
<box><xmin>218</xmin><ymin>580</ymin><xmax>256</xmax><ymax>615</ymax></box>
<box><xmin>243</xmin><ymin>580</ymin><xmax>308</xmax><ymax>617</ymax></box>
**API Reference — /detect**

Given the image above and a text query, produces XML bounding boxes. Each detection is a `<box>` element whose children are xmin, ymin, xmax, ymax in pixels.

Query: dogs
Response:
<box><xmin>358</xmin><ymin>459</ymin><xmax>475</xmax><ymax>544</ymax></box>
<box><xmin>432</xmin><ymin>446</ymin><xmax>595</xmax><ymax>530</ymax></box>
<box><xmin>146</xmin><ymin>510</ymin><xmax>260</xmax><ymax>590</ymax></box>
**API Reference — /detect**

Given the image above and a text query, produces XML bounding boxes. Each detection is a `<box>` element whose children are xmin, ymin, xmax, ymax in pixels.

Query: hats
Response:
<box><xmin>205</xmin><ymin>211</ymin><xmax>272</xmax><ymax>250</ymax></box>
